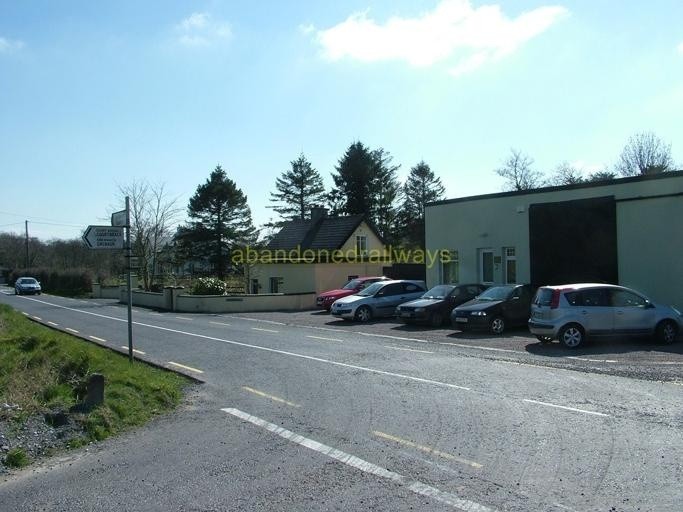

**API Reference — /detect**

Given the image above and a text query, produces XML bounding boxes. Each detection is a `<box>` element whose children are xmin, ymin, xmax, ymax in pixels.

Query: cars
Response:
<box><xmin>449</xmin><ymin>283</ymin><xmax>546</xmax><ymax>336</ymax></box>
<box><xmin>317</xmin><ymin>275</ymin><xmax>397</xmax><ymax>314</ymax></box>
<box><xmin>329</xmin><ymin>279</ymin><xmax>429</xmax><ymax>325</ymax></box>
<box><xmin>14</xmin><ymin>276</ymin><xmax>44</xmax><ymax>298</ymax></box>
<box><xmin>392</xmin><ymin>282</ymin><xmax>491</xmax><ymax>330</ymax></box>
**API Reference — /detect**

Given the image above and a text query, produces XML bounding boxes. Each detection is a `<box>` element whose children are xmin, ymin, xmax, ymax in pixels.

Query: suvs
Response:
<box><xmin>528</xmin><ymin>282</ymin><xmax>683</xmax><ymax>350</ymax></box>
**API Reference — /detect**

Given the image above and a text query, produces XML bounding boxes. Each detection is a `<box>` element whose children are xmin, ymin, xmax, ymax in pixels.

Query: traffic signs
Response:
<box><xmin>129</xmin><ymin>255</ymin><xmax>149</xmax><ymax>270</ymax></box>
<box><xmin>111</xmin><ymin>209</ymin><xmax>129</xmax><ymax>229</ymax></box>
<box><xmin>83</xmin><ymin>225</ymin><xmax>128</xmax><ymax>252</ymax></box>
